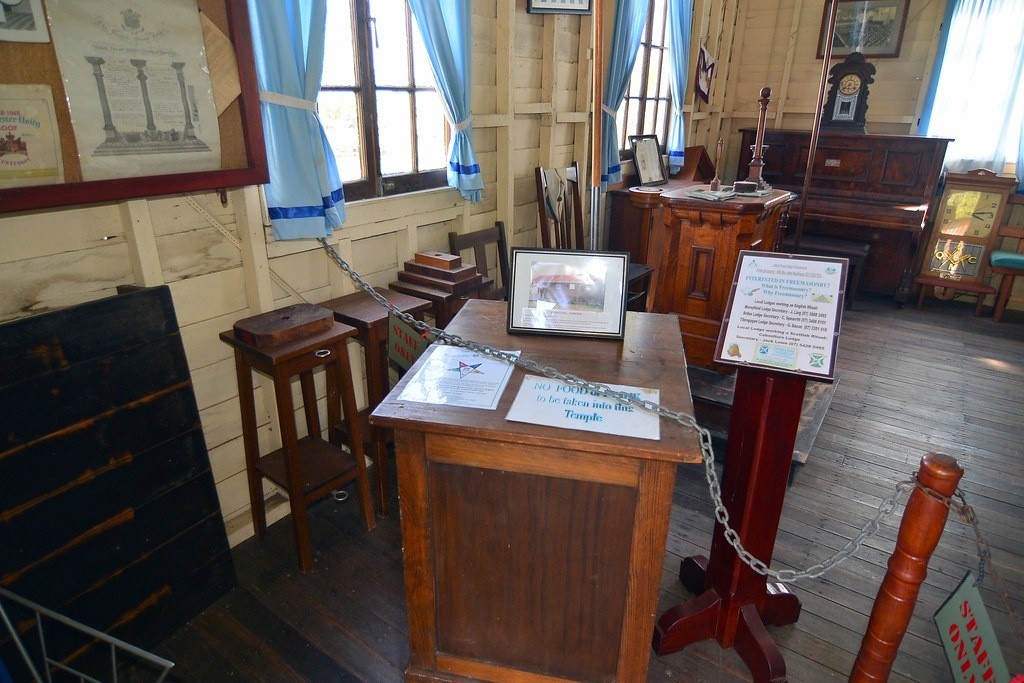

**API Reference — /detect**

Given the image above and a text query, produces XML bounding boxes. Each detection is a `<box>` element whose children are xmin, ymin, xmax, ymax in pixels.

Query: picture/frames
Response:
<box><xmin>506</xmin><ymin>247</ymin><xmax>630</xmax><ymax>339</ymax></box>
<box><xmin>816</xmin><ymin>0</ymin><xmax>911</xmax><ymax>59</ymax></box>
<box><xmin>628</xmin><ymin>135</ymin><xmax>668</xmax><ymax>186</ymax></box>
<box><xmin>527</xmin><ymin>0</ymin><xmax>592</xmax><ymax>14</ymax></box>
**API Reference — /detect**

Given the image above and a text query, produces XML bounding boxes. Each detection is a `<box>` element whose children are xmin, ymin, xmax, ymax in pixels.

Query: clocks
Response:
<box><xmin>819</xmin><ymin>52</ymin><xmax>876</xmax><ymax>135</ymax></box>
<box><xmin>912</xmin><ymin>168</ymin><xmax>1019</xmax><ymax>318</ymax></box>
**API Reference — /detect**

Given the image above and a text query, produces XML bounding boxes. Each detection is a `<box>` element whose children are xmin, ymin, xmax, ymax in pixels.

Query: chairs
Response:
<box><xmin>448</xmin><ymin>221</ymin><xmax>509</xmax><ymax>302</ymax></box>
<box><xmin>535</xmin><ymin>161</ymin><xmax>655</xmax><ymax>314</ymax></box>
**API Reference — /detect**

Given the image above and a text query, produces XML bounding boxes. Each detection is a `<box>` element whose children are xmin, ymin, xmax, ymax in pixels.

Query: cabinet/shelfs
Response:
<box><xmin>369</xmin><ymin>298</ymin><xmax>703</xmax><ymax>683</ymax></box>
<box><xmin>316</xmin><ymin>286</ymin><xmax>432</xmax><ymax>519</ymax></box>
<box><xmin>389</xmin><ymin>251</ymin><xmax>494</xmax><ymax>338</ymax></box>
<box><xmin>650</xmin><ymin>184</ymin><xmax>799</xmax><ymax>375</ymax></box>
<box><xmin>219</xmin><ymin>303</ymin><xmax>376</xmax><ymax>574</ymax></box>
<box><xmin>610</xmin><ymin>180</ymin><xmax>704</xmax><ymax>265</ymax></box>
<box><xmin>738</xmin><ymin>128</ymin><xmax>955</xmax><ymax>309</ymax></box>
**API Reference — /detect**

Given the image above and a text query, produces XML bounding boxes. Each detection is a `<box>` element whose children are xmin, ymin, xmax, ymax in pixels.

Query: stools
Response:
<box><xmin>784</xmin><ymin>233</ymin><xmax>874</xmax><ymax>310</ymax></box>
